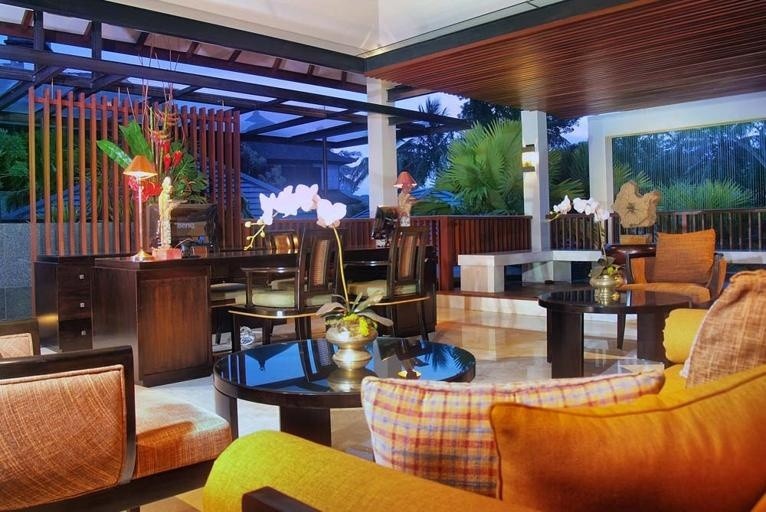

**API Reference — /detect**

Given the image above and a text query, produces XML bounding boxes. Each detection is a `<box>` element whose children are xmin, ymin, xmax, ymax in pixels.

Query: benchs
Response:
<box><xmin>457</xmin><ymin>249</ymin><xmax>766</xmax><ymax>293</ymax></box>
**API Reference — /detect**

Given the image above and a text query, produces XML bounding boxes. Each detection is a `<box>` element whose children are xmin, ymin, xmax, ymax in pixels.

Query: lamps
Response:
<box><xmin>122</xmin><ymin>154</ymin><xmax>159</xmax><ymax>262</ymax></box>
<box><xmin>392</xmin><ymin>171</ymin><xmax>418</xmax><ymax>226</ymax></box>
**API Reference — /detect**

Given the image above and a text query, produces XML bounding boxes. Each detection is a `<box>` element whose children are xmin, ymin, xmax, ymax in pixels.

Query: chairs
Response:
<box><xmin>601</xmin><ymin>245</ymin><xmax>727</xmax><ymax>349</ymax></box>
<box><xmin>347</xmin><ymin>225</ymin><xmax>430</xmax><ymax>341</ymax></box>
<box><xmin>254</xmin><ymin>229</ymin><xmax>333</xmax><ymax>293</ymax></box>
<box><xmin>0</xmin><ymin>317</ymin><xmax>234</xmax><ymax>512</ymax></box>
<box><xmin>179</xmin><ymin>240</ymin><xmax>256</xmax><ymax>346</ymax></box>
<box><xmin>298</xmin><ymin>338</ymin><xmax>342</xmax><ymax>384</ymax></box>
<box><xmin>377</xmin><ymin>337</ymin><xmax>433</xmax><ymax>361</ymax></box>
<box><xmin>228</xmin><ymin>226</ymin><xmax>349</xmax><ymax>353</ymax></box>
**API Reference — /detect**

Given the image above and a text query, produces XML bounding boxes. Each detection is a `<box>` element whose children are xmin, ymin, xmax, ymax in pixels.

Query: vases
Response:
<box><xmin>589</xmin><ymin>267</ymin><xmax>623</xmax><ymax>303</ymax></box>
<box><xmin>325</xmin><ymin>324</ymin><xmax>379</xmax><ymax>362</ymax></box>
<box><xmin>327</xmin><ymin>358</ymin><xmax>379</xmax><ymax>393</ymax></box>
<box><xmin>589</xmin><ymin>274</ymin><xmax>620</xmax><ymax>298</ymax></box>
<box><xmin>158</xmin><ymin>194</ymin><xmax>172</xmax><ymax>247</ymax></box>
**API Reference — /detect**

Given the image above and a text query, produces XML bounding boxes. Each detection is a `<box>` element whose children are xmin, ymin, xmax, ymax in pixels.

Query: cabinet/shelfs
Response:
<box><xmin>33</xmin><ymin>247</ymin><xmax>288</xmax><ymax>387</ymax></box>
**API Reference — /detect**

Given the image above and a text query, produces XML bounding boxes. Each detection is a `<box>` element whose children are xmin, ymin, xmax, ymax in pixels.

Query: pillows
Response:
<box><xmin>678</xmin><ymin>268</ymin><xmax>766</xmax><ymax>389</ymax></box>
<box><xmin>652</xmin><ymin>229</ymin><xmax>716</xmax><ymax>282</ymax></box>
<box><xmin>488</xmin><ymin>364</ymin><xmax>766</xmax><ymax>512</ymax></box>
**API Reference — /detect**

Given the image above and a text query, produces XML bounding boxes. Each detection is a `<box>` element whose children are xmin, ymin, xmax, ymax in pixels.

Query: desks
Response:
<box><xmin>88</xmin><ymin>245</ymin><xmax>439</xmax><ymax>389</ymax></box>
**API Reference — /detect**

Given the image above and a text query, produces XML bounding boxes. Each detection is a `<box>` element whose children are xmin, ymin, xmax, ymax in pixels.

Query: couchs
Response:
<box><xmin>202</xmin><ymin>307</ymin><xmax>766</xmax><ymax>512</ymax></box>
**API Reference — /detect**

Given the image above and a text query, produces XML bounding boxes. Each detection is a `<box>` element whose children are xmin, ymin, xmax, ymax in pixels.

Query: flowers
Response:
<box><xmin>96</xmin><ymin>107</ymin><xmax>210</xmax><ymax>209</ymax></box>
<box><xmin>548</xmin><ymin>194</ymin><xmax>627</xmax><ymax>279</ymax></box>
<box><xmin>243</xmin><ymin>183</ymin><xmax>394</xmax><ymax>337</ymax></box>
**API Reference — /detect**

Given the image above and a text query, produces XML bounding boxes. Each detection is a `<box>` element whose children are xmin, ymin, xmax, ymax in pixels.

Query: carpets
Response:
<box><xmin>139</xmin><ymin>319</ymin><xmax>637</xmax><ymax>512</ymax></box>
